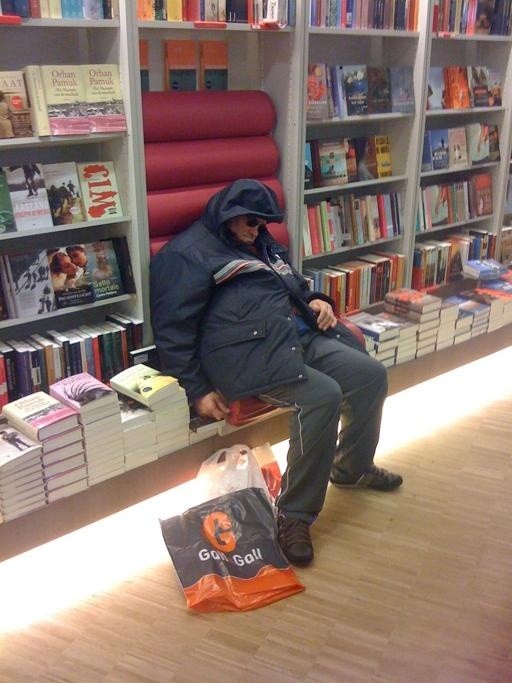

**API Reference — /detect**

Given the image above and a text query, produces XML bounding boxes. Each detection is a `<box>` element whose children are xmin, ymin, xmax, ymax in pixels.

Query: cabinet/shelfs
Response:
<box><xmin>1</xmin><ymin>1</ymin><xmax>511</xmax><ymax>347</ymax></box>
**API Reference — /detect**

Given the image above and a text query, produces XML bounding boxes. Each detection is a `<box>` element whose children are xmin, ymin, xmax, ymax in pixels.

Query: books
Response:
<box><xmin>432</xmin><ymin>0</ymin><xmax>512</xmax><ymax>36</ymax></box>
<box><xmin>413</xmin><ymin>66</ymin><xmax>512</xmax><ymax>289</ymax></box>
<box><xmin>348</xmin><ymin>260</ymin><xmax>512</xmax><ymax>367</ymax></box>
<box><xmin>303</xmin><ymin>64</ymin><xmax>405</xmax><ymax>311</ymax></box>
<box><xmin>189</xmin><ymin>403</ymin><xmax>296</xmax><ymax>447</ymax></box>
<box><xmin>137</xmin><ymin>0</ymin><xmax>296</xmax><ymax>25</ymax></box>
<box><xmin>1</xmin><ymin>65</ymin><xmax>190</xmax><ymax>523</ymax></box>
<box><xmin>0</xmin><ymin>0</ymin><xmax>120</xmax><ymax>20</ymax></box>
<box><xmin>311</xmin><ymin>0</ymin><xmax>418</xmax><ymax>33</ymax></box>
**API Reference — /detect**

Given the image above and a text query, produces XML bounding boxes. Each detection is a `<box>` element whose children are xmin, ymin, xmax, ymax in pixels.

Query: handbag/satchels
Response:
<box><xmin>156</xmin><ymin>444</ymin><xmax>306</xmax><ymax>614</ymax></box>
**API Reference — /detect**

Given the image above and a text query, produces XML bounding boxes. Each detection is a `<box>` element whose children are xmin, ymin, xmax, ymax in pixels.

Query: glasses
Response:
<box><xmin>244</xmin><ymin>216</ymin><xmax>267</xmax><ymax>234</ymax></box>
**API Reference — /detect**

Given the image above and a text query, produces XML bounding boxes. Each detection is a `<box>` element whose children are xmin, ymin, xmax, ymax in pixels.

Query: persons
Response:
<box><xmin>150</xmin><ymin>179</ymin><xmax>403</xmax><ymax>564</ymax></box>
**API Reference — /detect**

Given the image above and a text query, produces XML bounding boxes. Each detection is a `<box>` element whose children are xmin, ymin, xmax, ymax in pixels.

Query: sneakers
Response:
<box><xmin>327</xmin><ymin>463</ymin><xmax>403</xmax><ymax>491</ymax></box>
<box><xmin>277</xmin><ymin>506</ymin><xmax>314</xmax><ymax>567</ymax></box>
